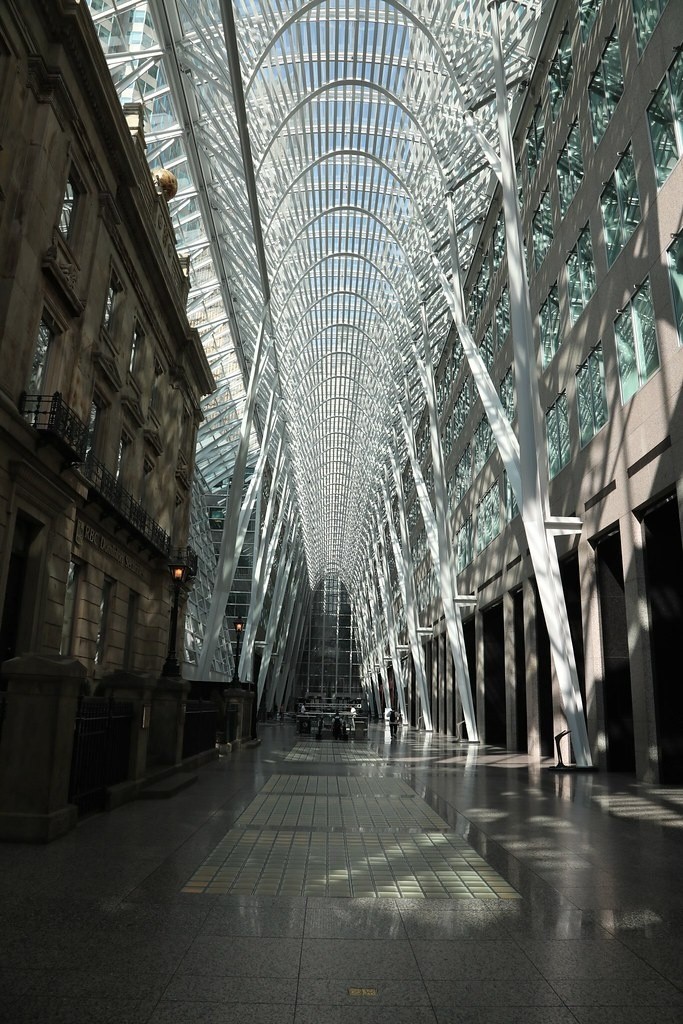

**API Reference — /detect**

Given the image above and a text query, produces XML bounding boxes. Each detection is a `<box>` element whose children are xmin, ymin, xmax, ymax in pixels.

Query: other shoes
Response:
<box><xmin>390</xmin><ymin>737</ymin><xmax>393</xmax><ymax>739</ymax></box>
<box><xmin>393</xmin><ymin>733</ymin><xmax>397</xmax><ymax>738</ymax></box>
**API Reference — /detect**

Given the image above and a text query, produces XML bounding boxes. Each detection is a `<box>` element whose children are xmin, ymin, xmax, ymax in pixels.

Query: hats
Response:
<box><xmin>391</xmin><ymin>706</ymin><xmax>395</xmax><ymax>709</ymax></box>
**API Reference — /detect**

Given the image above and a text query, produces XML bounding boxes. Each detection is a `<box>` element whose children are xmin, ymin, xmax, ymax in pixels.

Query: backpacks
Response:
<box><xmin>390</xmin><ymin>710</ymin><xmax>396</xmax><ymax>722</ymax></box>
<box><xmin>333</xmin><ymin>717</ymin><xmax>341</xmax><ymax>728</ymax></box>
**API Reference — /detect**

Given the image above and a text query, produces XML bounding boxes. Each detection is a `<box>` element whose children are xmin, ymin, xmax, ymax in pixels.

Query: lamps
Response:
<box><xmin>232</xmin><ymin>615</ymin><xmax>245</xmax><ymax>632</ymax></box>
<box><xmin>167</xmin><ymin>549</ymin><xmax>192</xmax><ymax>584</ymax></box>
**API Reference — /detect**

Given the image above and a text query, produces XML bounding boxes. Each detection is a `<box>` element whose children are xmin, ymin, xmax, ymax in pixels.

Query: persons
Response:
<box><xmin>386</xmin><ymin>706</ymin><xmax>400</xmax><ymax>739</ymax></box>
<box><xmin>350</xmin><ymin>705</ymin><xmax>356</xmax><ymax>727</ymax></box>
<box><xmin>332</xmin><ymin>714</ymin><xmax>343</xmax><ymax>740</ymax></box>
<box><xmin>301</xmin><ymin>703</ymin><xmax>306</xmax><ymax>713</ymax></box>
